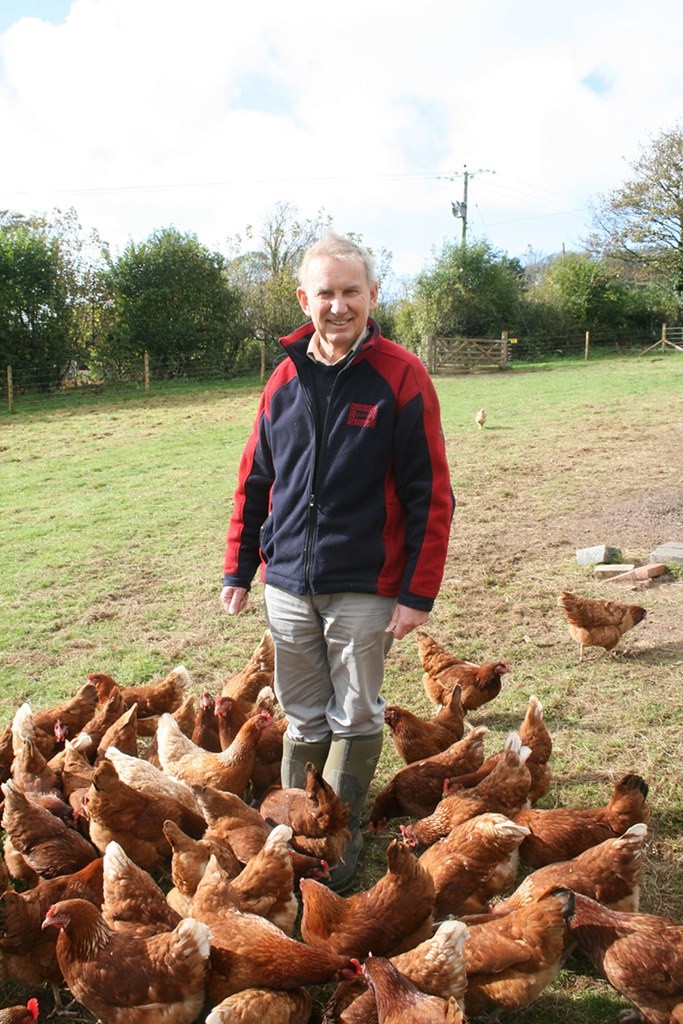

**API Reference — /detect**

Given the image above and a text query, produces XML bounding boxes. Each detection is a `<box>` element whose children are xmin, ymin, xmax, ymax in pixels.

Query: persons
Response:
<box><xmin>218</xmin><ymin>236</ymin><xmax>455</xmax><ymax>897</ymax></box>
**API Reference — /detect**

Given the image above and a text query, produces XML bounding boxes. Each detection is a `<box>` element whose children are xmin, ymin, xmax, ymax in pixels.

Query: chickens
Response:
<box><xmin>475</xmin><ymin>408</ymin><xmax>486</xmax><ymax>428</ymax></box>
<box><xmin>558</xmin><ymin>591</ymin><xmax>647</xmax><ymax>662</ymax></box>
<box><xmin>2</xmin><ymin>629</ymin><xmax>683</xmax><ymax>1024</ymax></box>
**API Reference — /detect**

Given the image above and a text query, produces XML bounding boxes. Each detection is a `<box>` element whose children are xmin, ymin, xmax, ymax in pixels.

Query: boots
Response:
<box><xmin>281</xmin><ymin>730</ymin><xmax>383</xmax><ymax>896</ymax></box>
